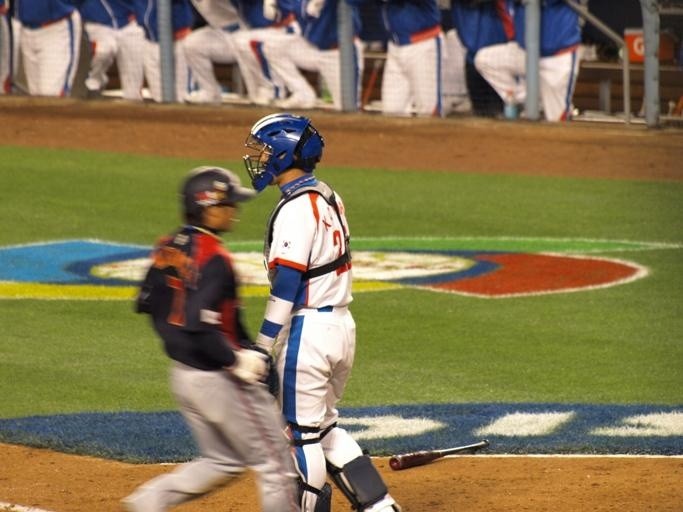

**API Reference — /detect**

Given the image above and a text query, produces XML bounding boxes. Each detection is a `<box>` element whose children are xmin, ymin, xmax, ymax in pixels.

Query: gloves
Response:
<box><xmin>225</xmin><ymin>349</ymin><xmax>273</xmax><ymax>385</ymax></box>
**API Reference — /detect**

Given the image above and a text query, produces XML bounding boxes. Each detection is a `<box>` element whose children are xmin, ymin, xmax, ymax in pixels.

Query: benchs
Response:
<box><xmin>348</xmin><ymin>51</ymin><xmax>683</xmax><ymax>118</ymax></box>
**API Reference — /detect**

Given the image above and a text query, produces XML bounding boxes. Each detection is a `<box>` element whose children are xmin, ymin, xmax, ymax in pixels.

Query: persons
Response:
<box><xmin>1</xmin><ymin>1</ymin><xmax>582</xmax><ymax>122</ymax></box>
<box><xmin>119</xmin><ymin>167</ymin><xmax>302</xmax><ymax>512</ymax></box>
<box><xmin>242</xmin><ymin>112</ymin><xmax>403</xmax><ymax>512</ymax></box>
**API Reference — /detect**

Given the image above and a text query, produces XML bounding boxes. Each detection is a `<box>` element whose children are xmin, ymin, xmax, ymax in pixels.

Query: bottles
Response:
<box><xmin>502</xmin><ymin>88</ymin><xmax>518</xmax><ymax>120</ymax></box>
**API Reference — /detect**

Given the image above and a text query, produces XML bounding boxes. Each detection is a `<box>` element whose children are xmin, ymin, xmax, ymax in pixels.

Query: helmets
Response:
<box><xmin>244</xmin><ymin>112</ymin><xmax>324</xmax><ymax>191</ymax></box>
<box><xmin>183</xmin><ymin>167</ymin><xmax>255</xmax><ymax>209</ymax></box>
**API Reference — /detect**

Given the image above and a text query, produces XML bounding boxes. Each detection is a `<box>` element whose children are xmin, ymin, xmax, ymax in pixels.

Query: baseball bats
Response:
<box><xmin>389</xmin><ymin>439</ymin><xmax>489</xmax><ymax>470</ymax></box>
